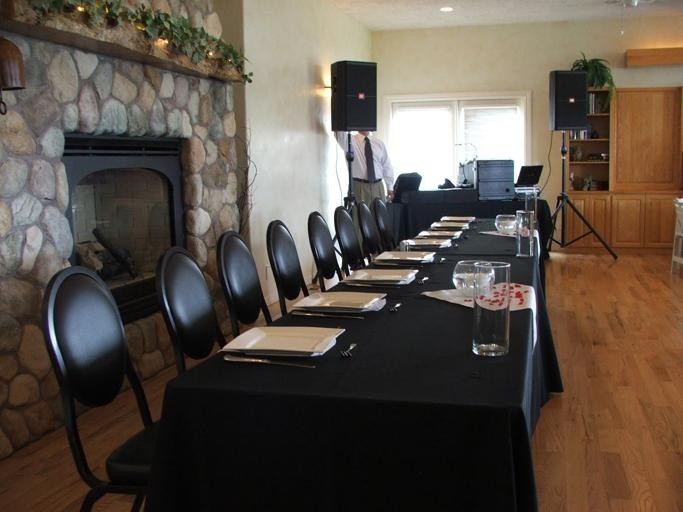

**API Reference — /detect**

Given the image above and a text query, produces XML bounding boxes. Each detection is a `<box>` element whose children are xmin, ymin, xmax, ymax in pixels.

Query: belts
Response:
<box><xmin>351</xmin><ymin>178</ymin><xmax>382</xmax><ymax>183</ymax></box>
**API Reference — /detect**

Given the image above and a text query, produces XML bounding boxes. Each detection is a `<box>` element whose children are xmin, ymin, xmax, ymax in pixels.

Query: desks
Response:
<box><xmin>407</xmin><ymin>189</ymin><xmax>551</xmax><ymax>259</ymax></box>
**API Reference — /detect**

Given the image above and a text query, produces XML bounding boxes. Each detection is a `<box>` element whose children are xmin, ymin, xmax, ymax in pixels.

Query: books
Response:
<box><xmin>593</xmin><ymin>93</ymin><xmax>595</xmax><ymax>114</ymax></box>
<box><xmin>588</xmin><ymin>93</ymin><xmax>593</xmax><ymax>114</ymax></box>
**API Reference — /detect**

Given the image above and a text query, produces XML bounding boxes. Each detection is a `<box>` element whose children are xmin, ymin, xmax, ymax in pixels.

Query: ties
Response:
<box><xmin>364</xmin><ymin>136</ymin><xmax>376</xmax><ymax>184</ymax></box>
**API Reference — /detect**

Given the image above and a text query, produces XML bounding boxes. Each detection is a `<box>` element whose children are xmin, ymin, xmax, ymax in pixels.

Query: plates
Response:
<box><xmin>222</xmin><ymin>216</ymin><xmax>474</xmax><ymax>355</ymax></box>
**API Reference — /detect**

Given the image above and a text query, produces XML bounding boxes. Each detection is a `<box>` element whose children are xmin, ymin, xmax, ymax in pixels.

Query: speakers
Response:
<box><xmin>549</xmin><ymin>70</ymin><xmax>587</xmax><ymax>132</ymax></box>
<box><xmin>331</xmin><ymin>61</ymin><xmax>377</xmax><ymax>131</ymax></box>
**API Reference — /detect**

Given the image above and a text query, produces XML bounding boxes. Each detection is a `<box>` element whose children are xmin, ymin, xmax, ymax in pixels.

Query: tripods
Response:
<box><xmin>312</xmin><ymin>131</ymin><xmax>371</xmax><ymax>284</ymax></box>
<box><xmin>546</xmin><ymin>131</ymin><xmax>618</xmax><ymax>260</ymax></box>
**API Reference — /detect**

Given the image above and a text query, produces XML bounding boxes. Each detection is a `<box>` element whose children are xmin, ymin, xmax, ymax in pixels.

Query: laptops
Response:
<box><xmin>514</xmin><ymin>166</ymin><xmax>543</xmax><ymax>187</ymax></box>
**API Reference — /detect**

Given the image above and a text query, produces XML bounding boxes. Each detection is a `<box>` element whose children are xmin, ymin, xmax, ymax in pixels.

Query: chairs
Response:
<box><xmin>374</xmin><ymin>196</ymin><xmax>397</xmax><ymax>251</ymax></box>
<box><xmin>334</xmin><ymin>206</ymin><xmax>366</xmax><ymax>276</ymax></box>
<box><xmin>356</xmin><ymin>199</ymin><xmax>386</xmax><ymax>263</ymax></box>
<box><xmin>42</xmin><ymin>266</ymin><xmax>161</xmax><ymax>512</ymax></box>
<box><xmin>307</xmin><ymin>212</ymin><xmax>343</xmax><ymax>292</ymax></box>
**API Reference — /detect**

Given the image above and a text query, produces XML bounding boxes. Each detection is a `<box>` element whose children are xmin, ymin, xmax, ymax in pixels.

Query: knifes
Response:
<box><xmin>223</xmin><ymin>355</ymin><xmax>317</xmax><ymax>370</ymax></box>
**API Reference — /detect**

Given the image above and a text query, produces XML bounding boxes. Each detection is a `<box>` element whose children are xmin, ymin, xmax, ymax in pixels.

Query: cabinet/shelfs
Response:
<box><xmin>563</xmin><ymin>85</ymin><xmax>682</xmax><ymax>249</ymax></box>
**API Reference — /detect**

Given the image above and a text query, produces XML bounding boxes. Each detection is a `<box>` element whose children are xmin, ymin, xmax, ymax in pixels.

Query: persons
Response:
<box><xmin>334</xmin><ymin>131</ymin><xmax>394</xmax><ymax>269</ymax></box>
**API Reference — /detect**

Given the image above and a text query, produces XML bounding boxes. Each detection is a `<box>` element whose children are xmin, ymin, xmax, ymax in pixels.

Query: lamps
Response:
<box><xmin>0</xmin><ymin>34</ymin><xmax>26</xmax><ymax>115</ymax></box>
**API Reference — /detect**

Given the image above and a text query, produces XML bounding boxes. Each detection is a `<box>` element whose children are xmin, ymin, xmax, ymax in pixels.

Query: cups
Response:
<box><xmin>516</xmin><ymin>210</ymin><xmax>534</xmax><ymax>258</ymax></box>
<box><xmin>494</xmin><ymin>213</ymin><xmax>517</xmax><ymax>234</ymax></box>
<box><xmin>525</xmin><ymin>192</ymin><xmax>537</xmax><ymax>223</ymax></box>
<box><xmin>472</xmin><ymin>261</ymin><xmax>510</xmax><ymax>358</ymax></box>
<box><xmin>453</xmin><ymin>259</ymin><xmax>496</xmax><ymax>299</ymax></box>
